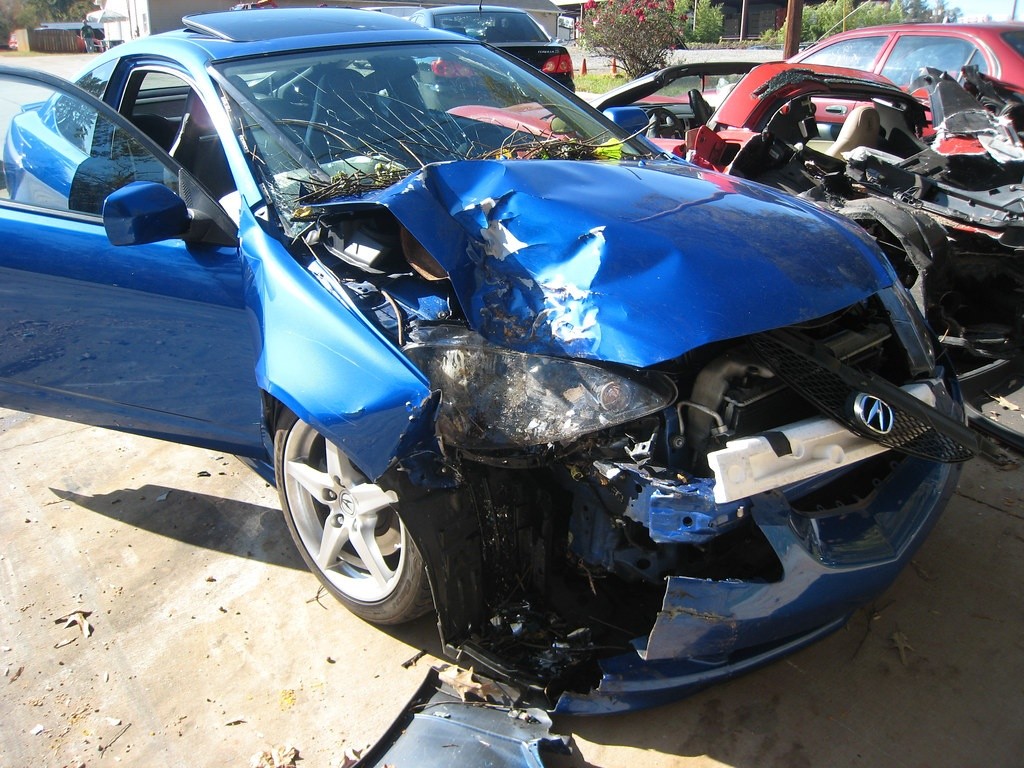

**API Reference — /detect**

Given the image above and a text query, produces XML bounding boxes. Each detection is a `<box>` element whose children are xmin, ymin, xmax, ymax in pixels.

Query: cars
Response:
<box><xmin>441</xmin><ymin>23</ymin><xmax>1024</xmax><ymax>403</ymax></box>
<box><xmin>8</xmin><ymin>33</ymin><xmax>18</xmax><ymax>51</ymax></box>
<box><xmin>1</xmin><ymin>6</ymin><xmax>969</xmax><ymax>723</ymax></box>
<box><xmin>406</xmin><ymin>4</ymin><xmax>577</xmax><ymax>103</ymax></box>
<box><xmin>360</xmin><ymin>5</ymin><xmax>425</xmax><ymax>17</ymax></box>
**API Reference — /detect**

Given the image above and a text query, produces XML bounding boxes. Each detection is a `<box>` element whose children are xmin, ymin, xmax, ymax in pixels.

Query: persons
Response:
<box><xmin>80</xmin><ymin>20</ymin><xmax>96</xmax><ymax>52</ymax></box>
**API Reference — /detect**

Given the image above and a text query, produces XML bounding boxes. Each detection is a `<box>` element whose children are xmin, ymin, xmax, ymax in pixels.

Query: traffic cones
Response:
<box><xmin>581</xmin><ymin>58</ymin><xmax>587</xmax><ymax>75</ymax></box>
<box><xmin>612</xmin><ymin>58</ymin><xmax>617</xmax><ymax>73</ymax></box>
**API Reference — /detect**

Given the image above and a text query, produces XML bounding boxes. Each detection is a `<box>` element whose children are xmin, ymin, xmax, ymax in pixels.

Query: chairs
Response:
<box><xmin>298</xmin><ymin>69</ymin><xmax>374</xmax><ymax>165</ymax></box>
<box><xmin>195</xmin><ymin>99</ymin><xmax>298</xmax><ymax>203</ymax></box>
<box><xmin>807</xmin><ymin>105</ymin><xmax>881</xmax><ymax>161</ymax></box>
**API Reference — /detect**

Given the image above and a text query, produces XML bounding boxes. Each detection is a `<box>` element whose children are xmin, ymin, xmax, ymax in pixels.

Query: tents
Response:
<box><xmin>87</xmin><ymin>10</ymin><xmax>127</xmax><ymax>49</ymax></box>
